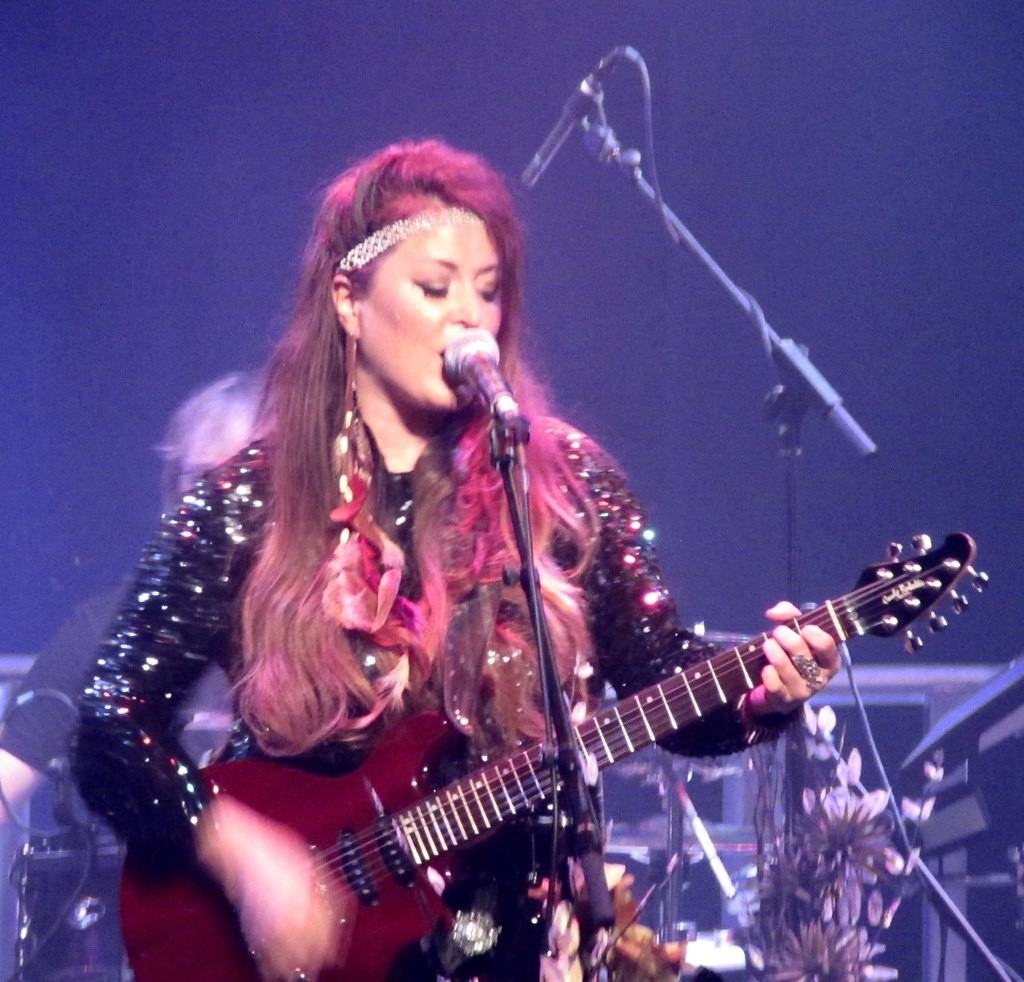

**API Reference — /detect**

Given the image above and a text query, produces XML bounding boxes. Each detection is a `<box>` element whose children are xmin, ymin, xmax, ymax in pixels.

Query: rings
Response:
<box><xmin>794</xmin><ymin>655</ymin><xmax>824</xmax><ymax>686</ymax></box>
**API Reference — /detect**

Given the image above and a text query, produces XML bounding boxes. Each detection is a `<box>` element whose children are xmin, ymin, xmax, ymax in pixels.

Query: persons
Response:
<box><xmin>77</xmin><ymin>143</ymin><xmax>841</xmax><ymax>982</ymax></box>
<box><xmin>1</xmin><ymin>366</ymin><xmax>272</xmax><ymax>982</ymax></box>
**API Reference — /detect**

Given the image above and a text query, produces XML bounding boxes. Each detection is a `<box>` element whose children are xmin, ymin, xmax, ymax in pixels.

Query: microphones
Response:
<box><xmin>521</xmin><ymin>47</ymin><xmax>622</xmax><ymax>188</ymax></box>
<box><xmin>443</xmin><ymin>329</ymin><xmax>526</xmax><ymax>443</ymax></box>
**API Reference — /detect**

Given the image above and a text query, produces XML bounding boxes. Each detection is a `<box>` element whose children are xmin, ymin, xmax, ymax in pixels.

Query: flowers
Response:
<box><xmin>762</xmin><ymin>700</ymin><xmax>946</xmax><ymax>982</ymax></box>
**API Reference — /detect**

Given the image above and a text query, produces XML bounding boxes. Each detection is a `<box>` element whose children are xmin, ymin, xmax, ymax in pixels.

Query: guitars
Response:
<box><xmin>119</xmin><ymin>530</ymin><xmax>991</xmax><ymax>982</ymax></box>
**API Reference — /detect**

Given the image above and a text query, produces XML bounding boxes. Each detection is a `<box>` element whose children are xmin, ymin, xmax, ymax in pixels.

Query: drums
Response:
<box><xmin>13</xmin><ymin>824</ymin><xmax>153</xmax><ymax>981</ymax></box>
<box><xmin>602</xmin><ymin>829</ymin><xmax>782</xmax><ymax>975</ymax></box>
